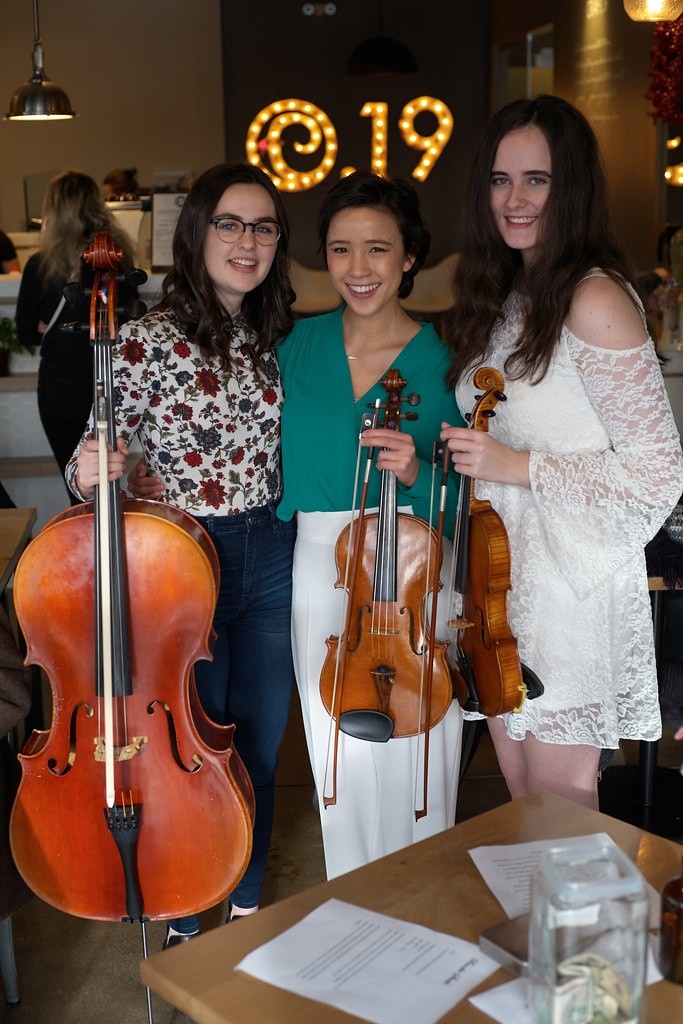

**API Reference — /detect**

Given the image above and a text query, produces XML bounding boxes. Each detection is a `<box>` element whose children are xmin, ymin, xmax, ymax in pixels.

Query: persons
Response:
<box><xmin>14</xmin><ymin>170</ymin><xmax>136</xmax><ymax>509</ymax></box>
<box><xmin>126</xmin><ymin>168</ymin><xmax>466</xmax><ymax>886</ymax></box>
<box><xmin>65</xmin><ymin>162</ymin><xmax>301</xmax><ymax>950</ymax></box>
<box><xmin>102</xmin><ymin>168</ymin><xmax>140</xmax><ymax>202</ymax></box>
<box><xmin>444</xmin><ymin>89</ymin><xmax>683</xmax><ymax>811</ymax></box>
<box><xmin>0</xmin><ymin>230</ymin><xmax>21</xmax><ymax>274</ymax></box>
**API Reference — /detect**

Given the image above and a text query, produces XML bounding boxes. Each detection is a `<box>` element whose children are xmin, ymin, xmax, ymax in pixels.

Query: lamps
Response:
<box><xmin>624</xmin><ymin>0</ymin><xmax>683</xmax><ymax>23</ymax></box>
<box><xmin>2</xmin><ymin>0</ymin><xmax>77</xmax><ymax>122</ymax></box>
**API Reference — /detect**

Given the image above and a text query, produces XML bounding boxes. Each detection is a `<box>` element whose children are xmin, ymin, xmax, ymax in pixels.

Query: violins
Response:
<box><xmin>446</xmin><ymin>369</ymin><xmax>528</xmax><ymax>724</ymax></box>
<box><xmin>314</xmin><ymin>371</ymin><xmax>458</xmax><ymax>738</ymax></box>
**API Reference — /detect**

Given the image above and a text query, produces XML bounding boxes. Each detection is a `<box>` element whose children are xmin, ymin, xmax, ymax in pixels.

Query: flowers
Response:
<box><xmin>0</xmin><ymin>316</ymin><xmax>35</xmax><ymax>356</ymax></box>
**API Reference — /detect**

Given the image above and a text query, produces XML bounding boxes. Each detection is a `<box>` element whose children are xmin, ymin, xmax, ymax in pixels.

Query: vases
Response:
<box><xmin>0</xmin><ymin>347</ymin><xmax>10</xmax><ymax>377</ymax></box>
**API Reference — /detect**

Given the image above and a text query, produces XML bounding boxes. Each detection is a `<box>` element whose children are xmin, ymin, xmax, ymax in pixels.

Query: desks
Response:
<box><xmin>0</xmin><ymin>508</ymin><xmax>45</xmax><ymax>826</ymax></box>
<box><xmin>139</xmin><ymin>789</ymin><xmax>683</xmax><ymax>1024</ymax></box>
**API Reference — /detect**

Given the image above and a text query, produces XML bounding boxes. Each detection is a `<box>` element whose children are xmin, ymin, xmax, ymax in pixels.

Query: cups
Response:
<box><xmin>529</xmin><ymin>842</ymin><xmax>651</xmax><ymax>1024</ymax></box>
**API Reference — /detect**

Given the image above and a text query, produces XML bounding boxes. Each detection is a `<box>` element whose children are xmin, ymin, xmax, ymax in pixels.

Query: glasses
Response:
<box><xmin>208</xmin><ymin>217</ymin><xmax>281</xmax><ymax>246</ymax></box>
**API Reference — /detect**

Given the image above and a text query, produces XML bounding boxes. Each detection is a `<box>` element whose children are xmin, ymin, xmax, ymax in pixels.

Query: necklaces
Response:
<box><xmin>347</xmin><ymin>326</ymin><xmax>405</xmax><ymax>358</ymax></box>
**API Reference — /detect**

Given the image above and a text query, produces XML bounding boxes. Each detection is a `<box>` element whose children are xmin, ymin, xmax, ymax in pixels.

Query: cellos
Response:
<box><xmin>0</xmin><ymin>234</ymin><xmax>259</xmax><ymax>1024</ymax></box>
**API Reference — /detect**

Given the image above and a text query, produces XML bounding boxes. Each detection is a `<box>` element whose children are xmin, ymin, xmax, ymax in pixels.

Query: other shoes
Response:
<box><xmin>224</xmin><ymin>899</ymin><xmax>259</xmax><ymax>924</ymax></box>
<box><xmin>162</xmin><ymin>923</ymin><xmax>201</xmax><ymax>951</ymax></box>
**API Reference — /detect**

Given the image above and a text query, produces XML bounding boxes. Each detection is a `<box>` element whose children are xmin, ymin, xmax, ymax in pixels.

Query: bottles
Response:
<box><xmin>660</xmin><ymin>857</ymin><xmax>683</xmax><ymax>985</ymax></box>
